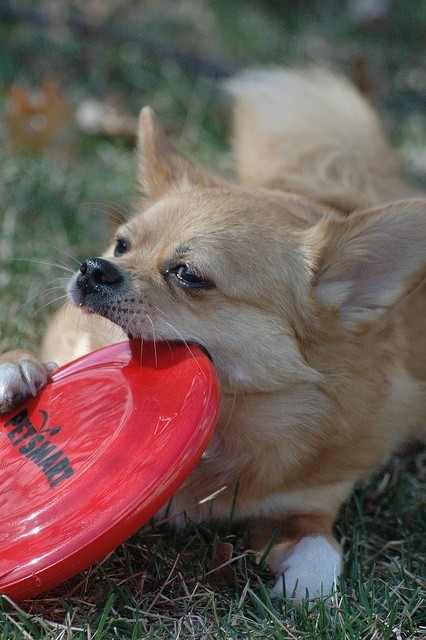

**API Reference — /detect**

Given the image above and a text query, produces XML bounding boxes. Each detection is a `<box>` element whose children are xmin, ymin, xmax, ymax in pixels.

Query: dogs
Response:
<box><xmin>0</xmin><ymin>61</ymin><xmax>426</xmax><ymax>612</ymax></box>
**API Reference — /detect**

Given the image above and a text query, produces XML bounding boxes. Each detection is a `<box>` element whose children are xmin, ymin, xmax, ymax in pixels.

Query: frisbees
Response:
<box><xmin>0</xmin><ymin>338</ymin><xmax>223</xmax><ymax>602</ymax></box>
